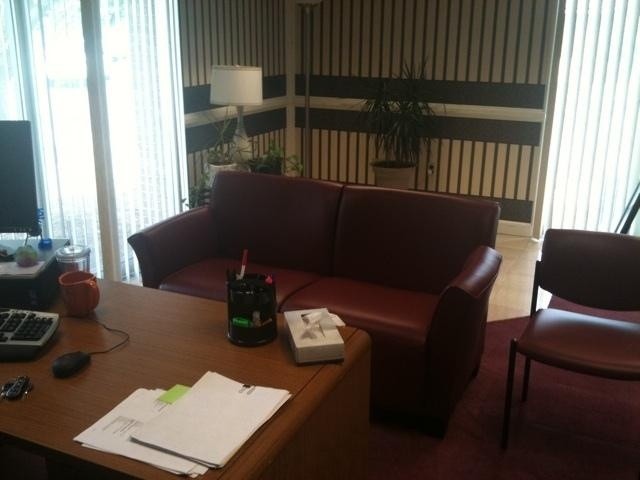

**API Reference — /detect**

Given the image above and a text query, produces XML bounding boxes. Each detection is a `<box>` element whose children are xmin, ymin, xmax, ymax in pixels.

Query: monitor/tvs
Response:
<box><xmin>0</xmin><ymin>120</ymin><xmax>39</xmax><ymax>262</ymax></box>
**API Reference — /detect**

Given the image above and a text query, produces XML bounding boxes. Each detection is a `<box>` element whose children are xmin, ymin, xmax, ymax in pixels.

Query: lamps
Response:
<box><xmin>208</xmin><ymin>62</ymin><xmax>266</xmax><ymax>162</ymax></box>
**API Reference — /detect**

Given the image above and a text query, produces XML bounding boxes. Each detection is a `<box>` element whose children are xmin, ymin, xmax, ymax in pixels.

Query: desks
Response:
<box><xmin>4</xmin><ymin>274</ymin><xmax>386</xmax><ymax>478</ymax></box>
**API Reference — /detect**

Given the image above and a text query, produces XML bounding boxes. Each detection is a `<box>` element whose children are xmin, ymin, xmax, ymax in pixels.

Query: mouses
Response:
<box><xmin>52</xmin><ymin>351</ymin><xmax>91</xmax><ymax>380</ymax></box>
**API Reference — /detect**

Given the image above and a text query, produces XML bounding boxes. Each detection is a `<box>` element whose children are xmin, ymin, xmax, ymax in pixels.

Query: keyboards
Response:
<box><xmin>0</xmin><ymin>307</ymin><xmax>61</xmax><ymax>354</ymax></box>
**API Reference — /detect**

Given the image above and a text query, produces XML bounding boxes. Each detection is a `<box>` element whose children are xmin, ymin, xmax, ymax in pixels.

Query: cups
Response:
<box><xmin>55</xmin><ymin>245</ymin><xmax>91</xmax><ymax>274</ymax></box>
<box><xmin>59</xmin><ymin>270</ymin><xmax>100</xmax><ymax>317</ymax></box>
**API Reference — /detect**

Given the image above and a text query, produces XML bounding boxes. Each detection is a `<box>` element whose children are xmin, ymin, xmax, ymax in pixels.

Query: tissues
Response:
<box><xmin>282</xmin><ymin>306</ymin><xmax>347</xmax><ymax>366</ymax></box>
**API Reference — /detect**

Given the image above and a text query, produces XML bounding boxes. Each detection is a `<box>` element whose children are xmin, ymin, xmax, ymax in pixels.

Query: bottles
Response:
<box><xmin>36</xmin><ymin>208</ymin><xmax>53</xmax><ymax>254</ymax></box>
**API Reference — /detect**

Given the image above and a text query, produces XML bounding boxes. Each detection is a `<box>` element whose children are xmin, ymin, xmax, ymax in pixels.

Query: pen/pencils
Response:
<box><xmin>240</xmin><ymin>249</ymin><xmax>248</xmax><ymax>280</ymax></box>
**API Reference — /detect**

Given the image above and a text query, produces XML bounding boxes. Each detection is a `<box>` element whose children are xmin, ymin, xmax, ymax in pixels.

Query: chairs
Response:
<box><xmin>499</xmin><ymin>227</ymin><xmax>637</xmax><ymax>454</ymax></box>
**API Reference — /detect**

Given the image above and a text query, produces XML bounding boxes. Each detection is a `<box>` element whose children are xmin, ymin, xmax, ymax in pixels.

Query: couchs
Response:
<box><xmin>128</xmin><ymin>169</ymin><xmax>504</xmax><ymax>443</ymax></box>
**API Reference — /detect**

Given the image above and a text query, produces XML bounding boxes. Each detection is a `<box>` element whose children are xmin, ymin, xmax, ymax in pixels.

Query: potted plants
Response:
<box><xmin>361</xmin><ymin>55</ymin><xmax>437</xmax><ymax>188</ymax></box>
<box><xmin>202</xmin><ymin>105</ymin><xmax>304</xmax><ymax>188</ymax></box>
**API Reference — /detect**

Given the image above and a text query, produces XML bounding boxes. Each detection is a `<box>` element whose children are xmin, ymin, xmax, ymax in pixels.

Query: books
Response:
<box><xmin>129</xmin><ymin>368</ymin><xmax>294</xmax><ymax>473</ymax></box>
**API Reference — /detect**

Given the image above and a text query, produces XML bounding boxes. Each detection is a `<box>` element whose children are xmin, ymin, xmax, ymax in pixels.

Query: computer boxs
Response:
<box><xmin>1</xmin><ymin>237</ymin><xmax>72</xmax><ymax>311</ymax></box>
